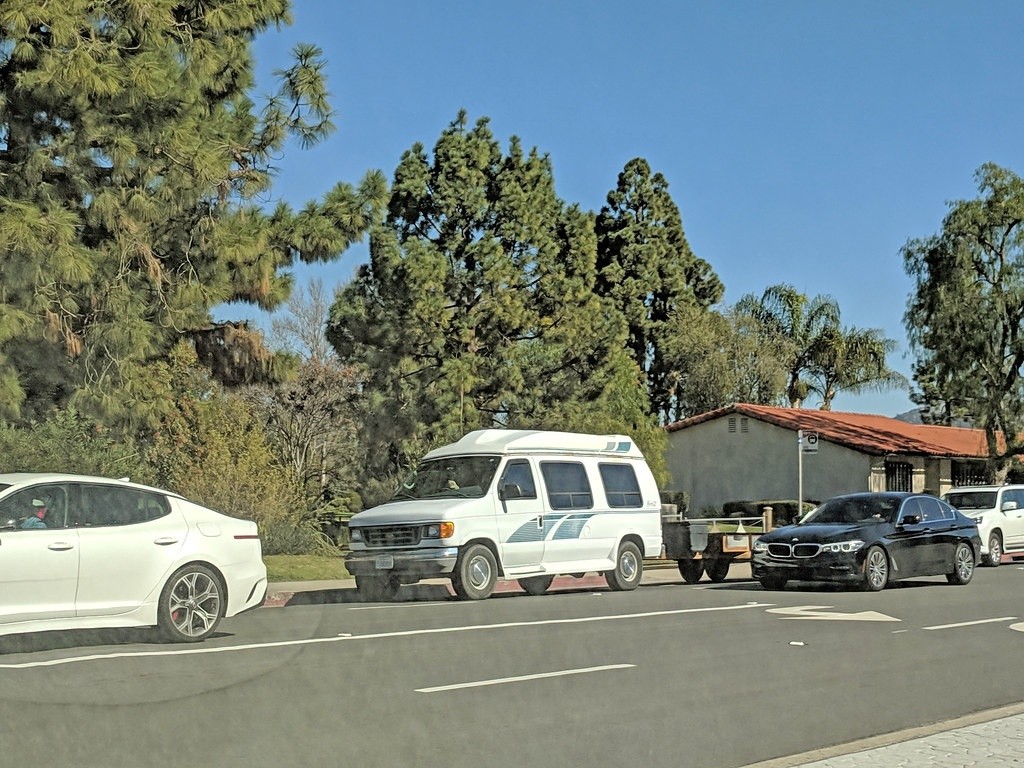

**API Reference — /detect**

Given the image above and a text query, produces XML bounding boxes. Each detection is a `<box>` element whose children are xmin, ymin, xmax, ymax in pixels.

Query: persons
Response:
<box><xmin>449</xmin><ymin>463</ymin><xmax>480</xmax><ymax>489</ymax></box>
<box><xmin>4</xmin><ymin>498</ymin><xmax>47</xmax><ymax>530</ymax></box>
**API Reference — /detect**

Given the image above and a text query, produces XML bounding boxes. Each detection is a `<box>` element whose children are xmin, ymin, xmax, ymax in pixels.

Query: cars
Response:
<box><xmin>0</xmin><ymin>470</ymin><xmax>268</xmax><ymax>650</ymax></box>
<box><xmin>752</xmin><ymin>492</ymin><xmax>981</xmax><ymax>596</ymax></box>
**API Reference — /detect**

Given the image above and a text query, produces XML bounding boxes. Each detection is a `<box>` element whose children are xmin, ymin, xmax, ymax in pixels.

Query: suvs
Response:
<box><xmin>937</xmin><ymin>484</ymin><xmax>1024</xmax><ymax>567</ymax></box>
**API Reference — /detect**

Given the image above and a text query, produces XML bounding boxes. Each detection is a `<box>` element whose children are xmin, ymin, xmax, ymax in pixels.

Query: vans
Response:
<box><xmin>346</xmin><ymin>425</ymin><xmax>663</xmax><ymax>602</ymax></box>
